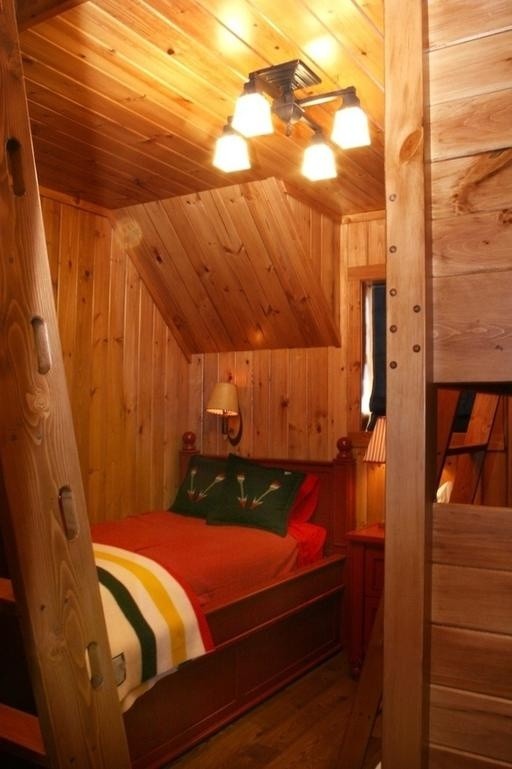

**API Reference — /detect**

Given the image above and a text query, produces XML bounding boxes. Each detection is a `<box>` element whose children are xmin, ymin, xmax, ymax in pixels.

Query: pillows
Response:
<box><xmin>290</xmin><ymin>470</ymin><xmax>321</xmax><ymax>539</ymax></box>
<box><xmin>208</xmin><ymin>452</ymin><xmax>302</xmax><ymax>540</ymax></box>
<box><xmin>169</xmin><ymin>453</ymin><xmax>225</xmax><ymax>518</ymax></box>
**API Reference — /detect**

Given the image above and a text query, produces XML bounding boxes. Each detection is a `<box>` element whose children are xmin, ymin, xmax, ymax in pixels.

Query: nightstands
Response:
<box><xmin>345</xmin><ymin>520</ymin><xmax>385</xmax><ymax>682</ymax></box>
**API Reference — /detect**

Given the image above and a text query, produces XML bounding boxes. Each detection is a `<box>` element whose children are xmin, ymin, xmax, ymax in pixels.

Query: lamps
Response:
<box><xmin>205</xmin><ymin>382</ymin><xmax>244</xmax><ymax>448</ymax></box>
<box><xmin>362</xmin><ymin>415</ymin><xmax>388</xmax><ymax>463</ymax></box>
<box><xmin>204</xmin><ymin>56</ymin><xmax>371</xmax><ymax>180</ymax></box>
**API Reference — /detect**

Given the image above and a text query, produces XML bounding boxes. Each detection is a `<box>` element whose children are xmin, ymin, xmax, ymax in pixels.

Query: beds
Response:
<box><xmin>0</xmin><ymin>425</ymin><xmax>360</xmax><ymax>767</ymax></box>
